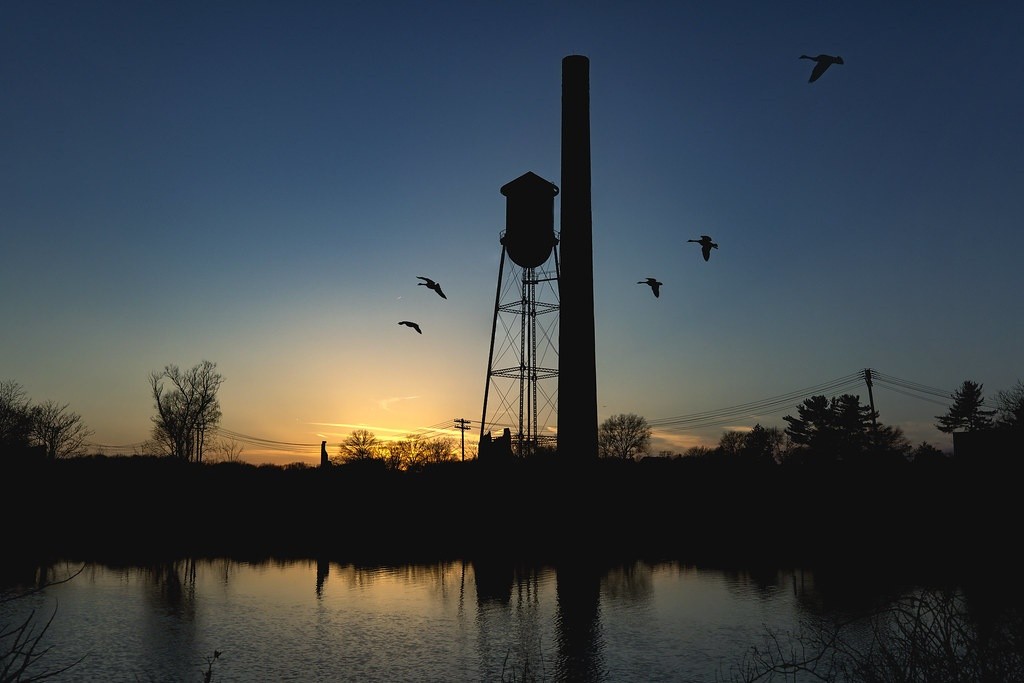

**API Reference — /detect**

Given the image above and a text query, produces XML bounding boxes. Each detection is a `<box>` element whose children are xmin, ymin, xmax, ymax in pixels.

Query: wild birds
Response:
<box><xmin>686</xmin><ymin>235</ymin><xmax>719</xmax><ymax>262</ymax></box>
<box><xmin>398</xmin><ymin>320</ymin><xmax>423</xmax><ymax>334</ymax></box>
<box><xmin>416</xmin><ymin>275</ymin><xmax>447</xmax><ymax>300</ymax></box>
<box><xmin>798</xmin><ymin>54</ymin><xmax>845</xmax><ymax>84</ymax></box>
<box><xmin>637</xmin><ymin>277</ymin><xmax>664</xmax><ymax>299</ymax></box>
